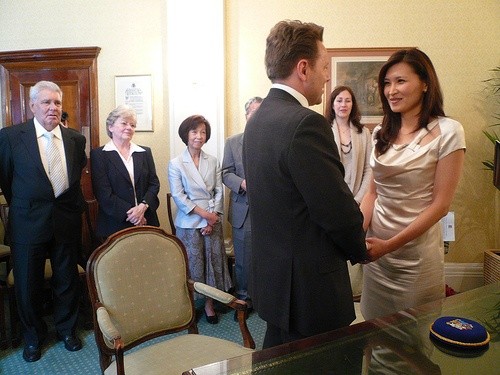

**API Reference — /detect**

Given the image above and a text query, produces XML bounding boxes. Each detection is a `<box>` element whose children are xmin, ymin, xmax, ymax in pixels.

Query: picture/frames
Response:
<box><xmin>323</xmin><ymin>47</ymin><xmax>418</xmax><ymax>134</ymax></box>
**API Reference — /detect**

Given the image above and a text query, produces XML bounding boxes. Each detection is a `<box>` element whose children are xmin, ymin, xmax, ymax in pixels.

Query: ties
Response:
<box><xmin>42</xmin><ymin>132</ymin><xmax>67</xmax><ymax>197</ymax></box>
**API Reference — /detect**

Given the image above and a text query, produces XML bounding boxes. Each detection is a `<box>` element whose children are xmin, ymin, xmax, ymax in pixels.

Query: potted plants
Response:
<box><xmin>481</xmin><ymin>67</ymin><xmax>500</xmax><ymax>285</ymax></box>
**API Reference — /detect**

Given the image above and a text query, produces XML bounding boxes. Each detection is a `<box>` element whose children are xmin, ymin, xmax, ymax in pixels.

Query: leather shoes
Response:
<box><xmin>22</xmin><ymin>343</ymin><xmax>42</xmax><ymax>363</ymax></box>
<box><xmin>55</xmin><ymin>329</ymin><xmax>83</xmax><ymax>351</ymax></box>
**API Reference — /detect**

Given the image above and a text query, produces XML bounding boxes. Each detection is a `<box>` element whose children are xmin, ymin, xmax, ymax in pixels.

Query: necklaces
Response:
<box><xmin>343</xmin><ymin>127</ymin><xmax>349</xmax><ymax>134</ymax></box>
<box><xmin>341</xmin><ymin>141</ymin><xmax>352</xmax><ymax>154</ymax></box>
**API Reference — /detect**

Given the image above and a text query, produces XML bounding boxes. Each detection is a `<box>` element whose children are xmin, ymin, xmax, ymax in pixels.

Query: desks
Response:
<box><xmin>182</xmin><ymin>279</ymin><xmax>500</xmax><ymax>375</ymax></box>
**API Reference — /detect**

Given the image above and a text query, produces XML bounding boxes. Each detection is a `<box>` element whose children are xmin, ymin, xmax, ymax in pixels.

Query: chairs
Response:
<box><xmin>86</xmin><ymin>225</ymin><xmax>255</xmax><ymax>375</ymax></box>
<box><xmin>0</xmin><ymin>199</ymin><xmax>86</xmax><ymax>347</ymax></box>
<box><xmin>167</xmin><ymin>192</ymin><xmax>176</xmax><ymax>235</ymax></box>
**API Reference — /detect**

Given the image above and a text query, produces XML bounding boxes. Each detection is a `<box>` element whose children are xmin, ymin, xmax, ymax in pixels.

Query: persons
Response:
<box><xmin>324</xmin><ymin>86</ymin><xmax>373</xmax><ymax>302</ymax></box>
<box><xmin>168</xmin><ymin>114</ymin><xmax>224</xmax><ymax>325</ymax></box>
<box><xmin>241</xmin><ymin>18</ymin><xmax>373</xmax><ymax>351</ymax></box>
<box><xmin>0</xmin><ymin>81</ymin><xmax>90</xmax><ymax>363</ymax></box>
<box><xmin>221</xmin><ymin>96</ymin><xmax>263</xmax><ymax>322</ymax></box>
<box><xmin>88</xmin><ymin>107</ymin><xmax>160</xmax><ymax>244</ymax></box>
<box><xmin>251</xmin><ymin>295</ymin><xmax>445</xmax><ymax>375</ymax></box>
<box><xmin>360</xmin><ymin>49</ymin><xmax>467</xmax><ymax>323</ymax></box>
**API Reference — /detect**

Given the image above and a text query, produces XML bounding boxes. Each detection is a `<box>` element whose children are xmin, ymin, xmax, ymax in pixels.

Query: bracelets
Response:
<box><xmin>142</xmin><ymin>202</ymin><xmax>149</xmax><ymax>208</ymax></box>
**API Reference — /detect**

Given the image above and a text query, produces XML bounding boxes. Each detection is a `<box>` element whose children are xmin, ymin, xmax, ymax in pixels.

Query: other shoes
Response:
<box><xmin>203</xmin><ymin>305</ymin><xmax>219</xmax><ymax>324</ymax></box>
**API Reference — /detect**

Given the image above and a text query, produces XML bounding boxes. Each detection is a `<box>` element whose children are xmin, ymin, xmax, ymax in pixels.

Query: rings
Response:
<box><xmin>133</xmin><ymin>218</ymin><xmax>135</xmax><ymax>219</ymax></box>
